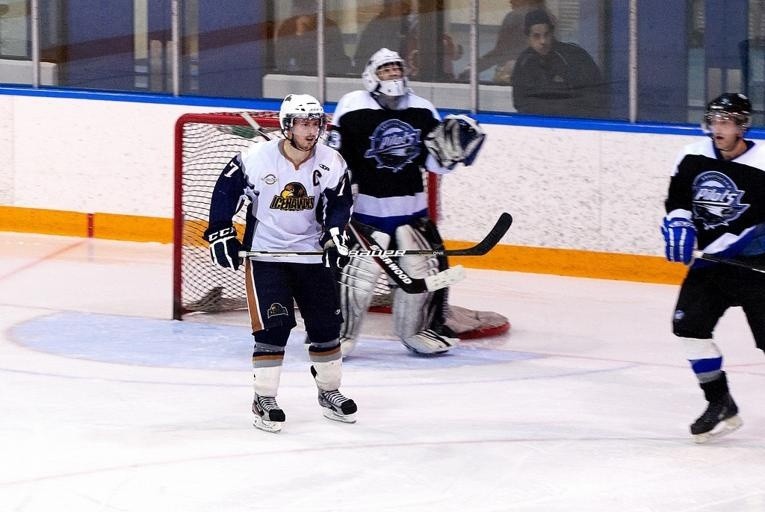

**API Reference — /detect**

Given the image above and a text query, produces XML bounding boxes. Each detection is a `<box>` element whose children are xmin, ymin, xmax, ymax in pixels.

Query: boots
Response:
<box><xmin>691</xmin><ymin>371</ymin><xmax>738</xmax><ymax>435</ymax></box>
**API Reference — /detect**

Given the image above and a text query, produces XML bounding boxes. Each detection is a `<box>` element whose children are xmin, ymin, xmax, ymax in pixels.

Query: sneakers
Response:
<box><xmin>252</xmin><ymin>392</ymin><xmax>285</xmax><ymax>421</ymax></box>
<box><xmin>318</xmin><ymin>388</ymin><xmax>357</xmax><ymax>415</ymax></box>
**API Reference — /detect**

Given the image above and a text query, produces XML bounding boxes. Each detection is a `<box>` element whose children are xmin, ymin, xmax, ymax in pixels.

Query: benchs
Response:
<box><xmin>0</xmin><ymin>58</ymin><xmax>61</xmax><ymax>86</ymax></box>
<box><xmin>261</xmin><ymin>70</ymin><xmax>516</xmax><ymax>114</ymax></box>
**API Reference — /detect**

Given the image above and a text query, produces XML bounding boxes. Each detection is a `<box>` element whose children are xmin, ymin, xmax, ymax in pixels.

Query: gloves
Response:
<box><xmin>318</xmin><ymin>227</ymin><xmax>350</xmax><ymax>269</ymax></box>
<box><xmin>662</xmin><ymin>208</ymin><xmax>699</xmax><ymax>265</ymax></box>
<box><xmin>202</xmin><ymin>227</ymin><xmax>244</xmax><ymax>271</ymax></box>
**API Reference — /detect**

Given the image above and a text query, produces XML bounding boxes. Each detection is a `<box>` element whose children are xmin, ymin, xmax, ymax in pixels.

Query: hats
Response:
<box><xmin>524</xmin><ymin>10</ymin><xmax>551</xmax><ymax>35</ymax></box>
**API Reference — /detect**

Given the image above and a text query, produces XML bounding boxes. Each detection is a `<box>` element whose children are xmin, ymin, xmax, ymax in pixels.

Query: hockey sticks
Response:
<box><xmin>241</xmin><ymin>112</ymin><xmax>468</xmax><ymax>293</ymax></box>
<box><xmin>237</xmin><ymin>213</ymin><xmax>512</xmax><ymax>257</ymax></box>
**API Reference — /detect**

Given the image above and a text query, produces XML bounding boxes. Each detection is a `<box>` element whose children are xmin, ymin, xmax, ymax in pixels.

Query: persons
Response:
<box><xmin>268</xmin><ymin>1</ymin><xmax>351</xmax><ymax>76</ymax></box>
<box><xmin>200</xmin><ymin>93</ymin><xmax>358</xmax><ymax>420</ymax></box>
<box><xmin>326</xmin><ymin>45</ymin><xmax>489</xmax><ymax>355</ymax></box>
<box><xmin>457</xmin><ymin>1</ymin><xmax>556</xmax><ymax>86</ymax></box>
<box><xmin>660</xmin><ymin>92</ymin><xmax>765</xmax><ymax>444</ymax></box>
<box><xmin>355</xmin><ymin>1</ymin><xmax>454</xmax><ymax>79</ymax></box>
<box><xmin>513</xmin><ymin>10</ymin><xmax>605</xmax><ymax>119</ymax></box>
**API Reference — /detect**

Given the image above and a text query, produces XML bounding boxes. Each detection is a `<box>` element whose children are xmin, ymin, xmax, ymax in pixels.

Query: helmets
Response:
<box><xmin>279</xmin><ymin>94</ymin><xmax>326</xmax><ymax>139</ymax></box>
<box><xmin>703</xmin><ymin>93</ymin><xmax>752</xmax><ymax>130</ymax></box>
<box><xmin>361</xmin><ymin>47</ymin><xmax>407</xmax><ymax>96</ymax></box>
